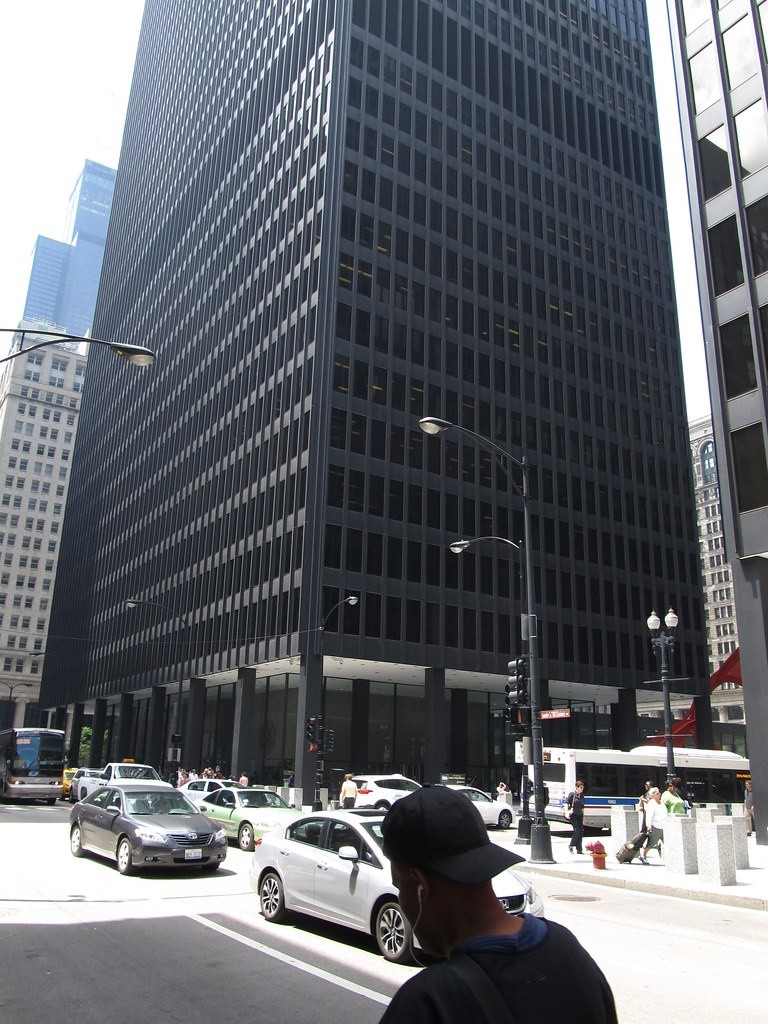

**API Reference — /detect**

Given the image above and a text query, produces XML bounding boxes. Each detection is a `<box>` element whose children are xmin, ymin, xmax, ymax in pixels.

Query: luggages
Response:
<box><xmin>615</xmin><ymin>830</ymin><xmax>651</xmax><ymax>864</ymax></box>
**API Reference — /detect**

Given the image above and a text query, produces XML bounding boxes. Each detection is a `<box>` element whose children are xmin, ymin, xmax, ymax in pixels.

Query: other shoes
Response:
<box><xmin>568</xmin><ymin>845</ymin><xmax>573</xmax><ymax>854</ymax></box>
<box><xmin>578</xmin><ymin>849</ymin><xmax>584</xmax><ymax>854</ymax></box>
<box><xmin>638</xmin><ymin>856</ymin><xmax>649</xmax><ymax>865</ymax></box>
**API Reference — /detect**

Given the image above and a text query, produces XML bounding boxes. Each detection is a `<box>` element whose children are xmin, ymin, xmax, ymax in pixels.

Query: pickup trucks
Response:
<box><xmin>77</xmin><ymin>763</ymin><xmax>174</xmax><ymax>802</ymax></box>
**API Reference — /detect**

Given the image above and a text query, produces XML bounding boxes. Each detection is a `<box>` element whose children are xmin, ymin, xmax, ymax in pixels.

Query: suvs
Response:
<box><xmin>339</xmin><ymin>774</ymin><xmax>423</xmax><ymax>811</ymax></box>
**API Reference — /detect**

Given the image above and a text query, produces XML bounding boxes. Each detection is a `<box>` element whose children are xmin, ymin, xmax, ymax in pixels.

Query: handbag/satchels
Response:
<box><xmin>637</xmin><ymin>795</ymin><xmax>646</xmax><ymax>810</ymax></box>
<box><xmin>563</xmin><ymin>792</ymin><xmax>575</xmax><ymax>820</ymax></box>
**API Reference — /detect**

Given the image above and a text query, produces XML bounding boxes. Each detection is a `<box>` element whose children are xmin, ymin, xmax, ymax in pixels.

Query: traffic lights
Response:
<box><xmin>324</xmin><ymin>729</ymin><xmax>336</xmax><ymax>752</ymax></box>
<box><xmin>510</xmin><ymin>707</ymin><xmax>528</xmax><ymax>725</ymax></box>
<box><xmin>306</xmin><ymin>714</ymin><xmax>319</xmax><ymax>742</ymax></box>
<box><xmin>507</xmin><ymin>658</ymin><xmax>528</xmax><ymax>704</ymax></box>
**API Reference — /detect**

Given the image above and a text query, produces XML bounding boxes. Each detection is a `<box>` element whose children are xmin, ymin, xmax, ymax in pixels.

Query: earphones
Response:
<box><xmin>417</xmin><ymin>883</ymin><xmax>423</xmax><ymax>902</ymax></box>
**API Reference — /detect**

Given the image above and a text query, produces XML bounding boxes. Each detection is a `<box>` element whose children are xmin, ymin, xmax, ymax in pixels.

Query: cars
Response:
<box><xmin>251</xmin><ymin>808</ymin><xmax>543</xmax><ymax>964</ymax></box>
<box><xmin>69</xmin><ymin>785</ymin><xmax>228</xmax><ymax>877</ymax></box>
<box><xmin>198</xmin><ymin>787</ymin><xmax>303</xmax><ymax>852</ymax></box>
<box><xmin>57</xmin><ymin>767</ymin><xmax>79</xmax><ymax>801</ymax></box>
<box><xmin>175</xmin><ymin>779</ymin><xmax>248</xmax><ymax>812</ymax></box>
<box><xmin>432</xmin><ymin>783</ymin><xmax>517</xmax><ymax>830</ymax></box>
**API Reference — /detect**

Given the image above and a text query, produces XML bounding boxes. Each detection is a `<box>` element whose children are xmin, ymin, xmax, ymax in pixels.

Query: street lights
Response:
<box><xmin>311</xmin><ymin>596</ymin><xmax>359</xmax><ymax>808</ymax></box>
<box><xmin>125</xmin><ymin>599</ymin><xmax>189</xmax><ymax>781</ymax></box>
<box><xmin>647</xmin><ymin>606</ymin><xmax>679</xmax><ymax>787</ymax></box>
<box><xmin>418</xmin><ymin>414</ymin><xmax>557</xmax><ymax>865</ymax></box>
<box><xmin>449</xmin><ymin>536</ymin><xmax>532</xmax><ymax>845</ymax></box>
<box><xmin>1</xmin><ymin>680</ymin><xmax>33</xmax><ymax>727</ymax></box>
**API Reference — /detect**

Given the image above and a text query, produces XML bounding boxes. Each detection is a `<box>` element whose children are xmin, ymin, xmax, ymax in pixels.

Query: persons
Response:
<box><xmin>637</xmin><ymin>786</ymin><xmax>666</xmax><ymax>864</ymax></box>
<box><xmin>287</xmin><ymin>772</ymin><xmax>295</xmax><ymax>788</ymax></box>
<box><xmin>672</xmin><ymin>777</ymin><xmax>688</xmax><ymax>801</ymax></box>
<box><xmin>743</xmin><ymin>780</ymin><xmax>755</xmax><ymax>836</ymax></box>
<box><xmin>339</xmin><ymin>774</ymin><xmax>357</xmax><ymax>809</ymax></box>
<box><xmin>496</xmin><ymin>782</ymin><xmax>506</xmax><ymax>796</ymax></box>
<box><xmin>175</xmin><ymin>766</ymin><xmax>224</xmax><ymax>788</ymax></box>
<box><xmin>640</xmin><ymin>780</ymin><xmax>656</xmax><ymax>833</ymax></box>
<box><xmin>660</xmin><ymin>780</ymin><xmax>685</xmax><ymax>813</ymax></box>
<box><xmin>565</xmin><ymin>781</ymin><xmax>585</xmax><ymax>854</ymax></box>
<box><xmin>238</xmin><ymin>772</ymin><xmax>248</xmax><ymax>786</ymax></box>
<box><xmin>380</xmin><ymin>781</ymin><xmax>620</xmax><ymax>1024</ymax></box>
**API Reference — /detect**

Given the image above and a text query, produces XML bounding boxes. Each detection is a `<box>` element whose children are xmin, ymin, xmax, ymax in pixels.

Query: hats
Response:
<box><xmin>381</xmin><ymin>782</ymin><xmax>525</xmax><ymax>888</ymax></box>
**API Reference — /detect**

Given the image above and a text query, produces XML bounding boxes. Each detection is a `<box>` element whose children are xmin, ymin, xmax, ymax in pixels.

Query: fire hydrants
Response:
<box><xmin>585</xmin><ymin>841</ymin><xmax>607</xmax><ymax>870</ymax></box>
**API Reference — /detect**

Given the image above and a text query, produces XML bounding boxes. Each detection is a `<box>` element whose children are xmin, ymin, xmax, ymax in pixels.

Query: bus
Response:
<box><xmin>521</xmin><ymin>745</ymin><xmax>753</xmax><ymax>837</ymax></box>
<box><xmin>0</xmin><ymin>727</ymin><xmax>72</xmax><ymax>806</ymax></box>
